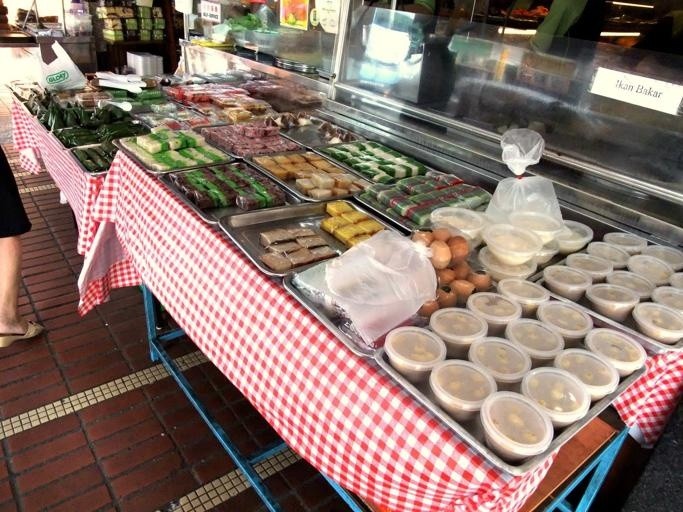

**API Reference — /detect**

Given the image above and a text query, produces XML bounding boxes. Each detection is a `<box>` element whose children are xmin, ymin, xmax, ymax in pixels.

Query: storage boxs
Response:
<box><xmin>126</xmin><ymin>51</ymin><xmax>164</xmax><ymax>77</ymax></box>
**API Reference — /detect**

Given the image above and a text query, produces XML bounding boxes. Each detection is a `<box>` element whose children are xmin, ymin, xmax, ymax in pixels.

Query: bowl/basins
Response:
<box><xmin>380</xmin><ymin>278</ymin><xmax>648</xmax><ymax>464</ymax></box>
<box><xmin>428</xmin><ymin>205</ymin><xmax>595</xmax><ymax>281</ymax></box>
<box><xmin>539</xmin><ymin>231</ymin><xmax>682</xmax><ymax>348</ymax></box>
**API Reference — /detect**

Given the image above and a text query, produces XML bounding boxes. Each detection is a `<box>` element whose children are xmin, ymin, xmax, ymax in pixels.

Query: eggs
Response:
<box><xmin>405</xmin><ymin>228</ymin><xmax>491</xmax><ymax>315</ymax></box>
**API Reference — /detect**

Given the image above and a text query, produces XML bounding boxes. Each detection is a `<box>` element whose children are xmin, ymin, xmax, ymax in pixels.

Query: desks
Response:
<box><xmin>1</xmin><ymin>27</ymin><xmax>98</xmax><ymax>77</ymax></box>
<box><xmin>5</xmin><ymin>73</ymin><xmax>330</xmax><ymax>318</ymax></box>
<box><xmin>77</xmin><ymin>119</ymin><xmax>683</xmax><ymax>512</ymax></box>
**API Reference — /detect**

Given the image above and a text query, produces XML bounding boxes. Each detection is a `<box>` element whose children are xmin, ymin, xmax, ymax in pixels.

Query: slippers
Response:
<box><xmin>1</xmin><ymin>320</ymin><xmax>45</xmax><ymax>348</ymax></box>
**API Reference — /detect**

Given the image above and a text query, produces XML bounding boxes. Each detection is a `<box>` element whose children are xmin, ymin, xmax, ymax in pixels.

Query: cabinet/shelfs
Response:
<box><xmin>86</xmin><ymin>0</ymin><xmax>179</xmax><ymax>73</ymax></box>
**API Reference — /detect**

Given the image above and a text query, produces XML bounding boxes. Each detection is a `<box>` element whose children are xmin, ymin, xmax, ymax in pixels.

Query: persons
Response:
<box><xmin>518</xmin><ymin>1</ymin><xmax>610</xmax><ymax>63</ymax></box>
<box><xmin>363</xmin><ymin>0</ymin><xmax>439</xmax><ymax>33</ymax></box>
<box><xmin>0</xmin><ymin>146</ymin><xmax>45</xmax><ymax>350</ymax></box>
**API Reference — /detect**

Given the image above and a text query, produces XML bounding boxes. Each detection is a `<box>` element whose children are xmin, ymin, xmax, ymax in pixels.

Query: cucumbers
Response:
<box><xmin>25</xmin><ymin>87</ymin><xmax>149</xmax><ymax>174</ymax></box>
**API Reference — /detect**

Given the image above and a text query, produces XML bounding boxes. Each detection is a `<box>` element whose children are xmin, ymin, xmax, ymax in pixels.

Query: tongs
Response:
<box><xmin>22</xmin><ymin>0</ymin><xmax>39</xmax><ymax>32</ymax></box>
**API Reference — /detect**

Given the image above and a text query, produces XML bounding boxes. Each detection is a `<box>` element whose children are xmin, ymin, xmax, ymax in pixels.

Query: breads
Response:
<box><xmin>259</xmin><ymin>227</ymin><xmax>338</xmax><ymax>271</ymax></box>
<box><xmin>322</xmin><ymin>201</ymin><xmax>383</xmax><ymax>249</ymax></box>
<box><xmin>252</xmin><ymin>153</ymin><xmax>369</xmax><ymax>200</ymax></box>
